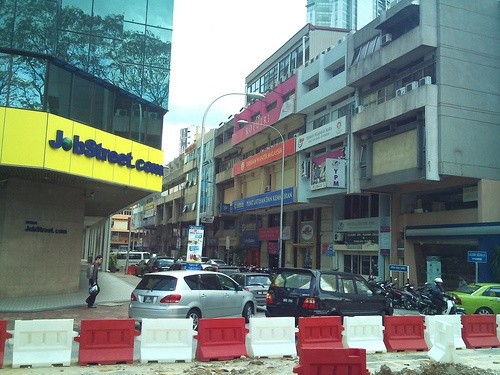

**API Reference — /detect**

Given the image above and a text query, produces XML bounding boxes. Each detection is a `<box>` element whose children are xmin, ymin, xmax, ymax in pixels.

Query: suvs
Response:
<box><xmin>266</xmin><ymin>267</ymin><xmax>394</xmax><ymax>330</ymax></box>
<box><xmin>202</xmin><ymin>265</ymin><xmax>240</xmax><ymax>288</ymax></box>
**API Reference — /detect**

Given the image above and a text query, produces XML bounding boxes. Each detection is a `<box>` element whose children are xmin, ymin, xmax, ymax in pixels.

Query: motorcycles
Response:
<box><xmin>377</xmin><ymin>278</ymin><xmax>466</xmax><ymax>320</ymax></box>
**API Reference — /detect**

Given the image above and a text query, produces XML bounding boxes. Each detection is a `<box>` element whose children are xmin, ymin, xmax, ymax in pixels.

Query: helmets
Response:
<box><xmin>434</xmin><ymin>278</ymin><xmax>443</xmax><ymax>286</ymax></box>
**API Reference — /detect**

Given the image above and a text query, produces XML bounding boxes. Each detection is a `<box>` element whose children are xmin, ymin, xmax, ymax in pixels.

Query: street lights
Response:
<box><xmin>194</xmin><ymin>92</ymin><xmax>267</xmax><ymax>228</ymax></box>
<box><xmin>238</xmin><ymin>120</ymin><xmax>285</xmax><ymax>270</ymax></box>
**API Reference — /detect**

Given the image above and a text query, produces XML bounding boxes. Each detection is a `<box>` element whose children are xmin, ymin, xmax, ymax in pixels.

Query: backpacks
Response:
<box><xmin>85</xmin><ymin>262</ymin><xmax>95</xmax><ymax>281</ymax></box>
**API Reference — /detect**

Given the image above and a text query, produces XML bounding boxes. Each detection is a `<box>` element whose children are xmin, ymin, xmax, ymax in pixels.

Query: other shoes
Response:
<box><xmin>85</xmin><ymin>300</ymin><xmax>96</xmax><ymax>308</ymax></box>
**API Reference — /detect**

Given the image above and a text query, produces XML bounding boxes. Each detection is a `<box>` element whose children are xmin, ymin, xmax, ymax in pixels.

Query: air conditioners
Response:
<box><xmin>395</xmin><ymin>86</ymin><xmax>406</xmax><ymax>95</ymax></box>
<box><xmin>380</xmin><ymin>32</ymin><xmax>392</xmax><ymax>47</ymax></box>
<box><xmin>419</xmin><ymin>76</ymin><xmax>431</xmax><ymax>86</ymax></box>
<box><xmin>405</xmin><ymin>80</ymin><xmax>418</xmax><ymax>92</ymax></box>
<box><xmin>333</xmin><ymin>233</ymin><xmax>344</xmax><ymax>243</ymax></box>
<box><xmin>263</xmin><ymin>185</ymin><xmax>271</xmax><ymax>194</ymax></box>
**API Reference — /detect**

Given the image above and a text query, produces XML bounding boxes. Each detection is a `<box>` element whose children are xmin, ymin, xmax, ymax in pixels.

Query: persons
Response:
<box><xmin>458</xmin><ymin>275</ymin><xmax>467</xmax><ymax>288</ymax></box>
<box><xmin>85</xmin><ymin>255</ymin><xmax>103</xmax><ymax>308</ymax></box>
<box><xmin>431</xmin><ymin>277</ymin><xmax>447</xmax><ymax>315</ymax></box>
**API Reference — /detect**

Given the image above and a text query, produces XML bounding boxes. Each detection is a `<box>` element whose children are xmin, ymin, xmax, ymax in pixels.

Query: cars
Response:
<box><xmin>448</xmin><ymin>282</ymin><xmax>500</xmax><ymax>315</ymax></box>
<box><xmin>128</xmin><ymin>270</ymin><xmax>256</xmax><ymax>331</ymax></box>
<box><xmin>146</xmin><ymin>256</ymin><xmax>227</xmax><ymax>271</ymax></box>
<box><xmin>230</xmin><ymin>272</ymin><xmax>274</xmax><ymax>308</ymax></box>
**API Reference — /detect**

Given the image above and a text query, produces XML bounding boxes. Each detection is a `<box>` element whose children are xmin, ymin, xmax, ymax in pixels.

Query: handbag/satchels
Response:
<box><xmin>89</xmin><ymin>284</ymin><xmax>100</xmax><ymax>295</ymax></box>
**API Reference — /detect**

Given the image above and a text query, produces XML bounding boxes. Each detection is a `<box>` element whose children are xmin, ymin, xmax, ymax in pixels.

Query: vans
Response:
<box><xmin>110</xmin><ymin>252</ymin><xmax>151</xmax><ymax>271</ymax></box>
